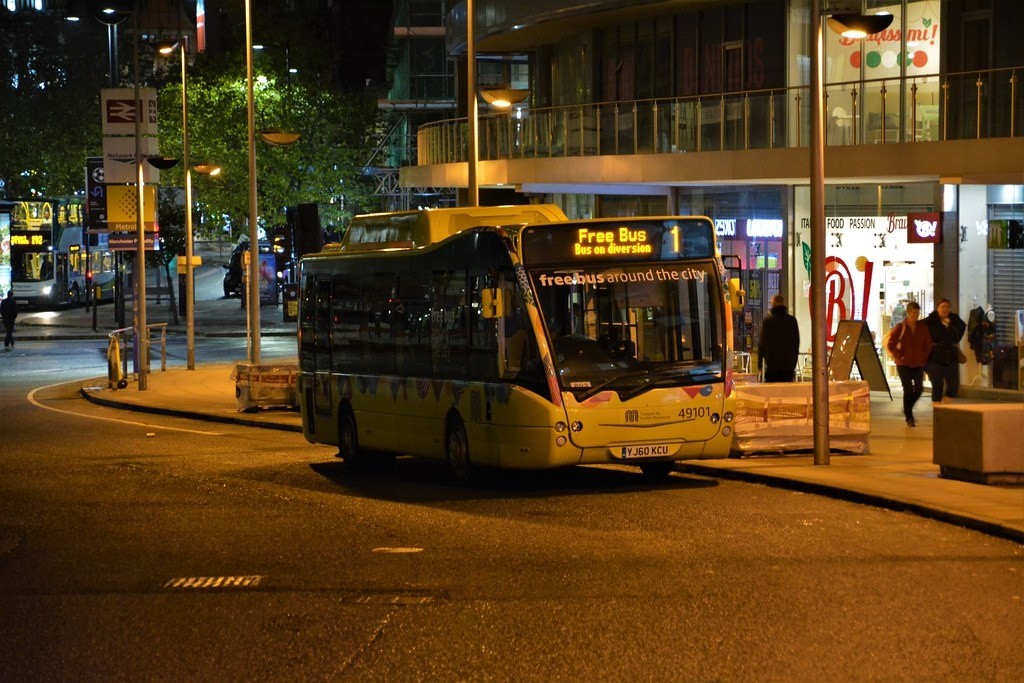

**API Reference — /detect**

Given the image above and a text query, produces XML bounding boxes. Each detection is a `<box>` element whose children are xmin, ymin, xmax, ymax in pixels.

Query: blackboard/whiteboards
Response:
<box><xmin>827</xmin><ymin>319</ymin><xmax>890</xmax><ymax>393</ymax></box>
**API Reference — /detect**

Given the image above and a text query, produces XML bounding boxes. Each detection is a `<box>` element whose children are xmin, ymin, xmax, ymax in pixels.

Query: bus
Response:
<box><xmin>297</xmin><ymin>202</ymin><xmax>746</xmax><ymax>489</ymax></box>
<box><xmin>1</xmin><ymin>194</ymin><xmax>134</xmax><ymax>310</ymax></box>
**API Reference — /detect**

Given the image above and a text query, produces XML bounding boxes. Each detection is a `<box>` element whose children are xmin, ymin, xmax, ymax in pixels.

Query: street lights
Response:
<box><xmin>160</xmin><ymin>41</ymin><xmax>218</xmax><ymax>371</ymax></box>
<box><xmin>468</xmin><ymin>87</ymin><xmax>532</xmax><ymax>207</ymax></box>
<box><xmin>97</xmin><ymin>1</ymin><xmax>178</xmax><ymax>392</ymax></box>
<box><xmin>248</xmin><ymin>131</ymin><xmax>303</xmax><ymax>363</ymax></box>
<box><xmin>810</xmin><ymin>9</ymin><xmax>895</xmax><ymax>467</ymax></box>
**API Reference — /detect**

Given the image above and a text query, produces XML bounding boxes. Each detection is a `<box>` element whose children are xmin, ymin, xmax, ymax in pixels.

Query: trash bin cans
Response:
<box><xmin>282</xmin><ymin>283</ymin><xmax>298</xmax><ymax>322</ymax></box>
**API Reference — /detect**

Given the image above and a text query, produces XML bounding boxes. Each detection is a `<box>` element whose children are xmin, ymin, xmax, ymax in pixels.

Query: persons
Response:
<box><xmin>38</xmin><ymin>255</ymin><xmax>53</xmax><ymax>281</ymax></box>
<box><xmin>921</xmin><ymin>299</ymin><xmax>968</xmax><ymax>403</ymax></box>
<box><xmin>888</xmin><ymin>302</ymin><xmax>932</xmax><ymax>426</ymax></box>
<box><xmin>756</xmin><ymin>296</ymin><xmax>800</xmax><ymax>383</ymax></box>
<box><xmin>0</xmin><ymin>290</ymin><xmax>18</xmax><ymax>351</ymax></box>
<box><xmin>43</xmin><ymin>211</ymin><xmax>52</xmax><ymax>225</ymax></box>
<box><xmin>11</xmin><ymin>215</ymin><xmax>22</xmax><ymax>231</ymax></box>
<box><xmin>968</xmin><ymin>296</ymin><xmax>995</xmax><ymax>364</ymax></box>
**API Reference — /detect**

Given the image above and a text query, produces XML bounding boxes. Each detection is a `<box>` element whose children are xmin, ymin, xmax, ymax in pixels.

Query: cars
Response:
<box><xmin>222</xmin><ymin>238</ymin><xmax>278</xmax><ymax>308</ymax></box>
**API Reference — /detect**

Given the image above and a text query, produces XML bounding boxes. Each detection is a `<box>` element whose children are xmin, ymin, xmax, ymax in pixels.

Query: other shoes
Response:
<box><xmin>5</xmin><ymin>346</ymin><xmax>10</xmax><ymax>352</ymax></box>
<box><xmin>11</xmin><ymin>344</ymin><xmax>15</xmax><ymax>349</ymax></box>
<box><xmin>906</xmin><ymin>416</ymin><xmax>915</xmax><ymax>427</ymax></box>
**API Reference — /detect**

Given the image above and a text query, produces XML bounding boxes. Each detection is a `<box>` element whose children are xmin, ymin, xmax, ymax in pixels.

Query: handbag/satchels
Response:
<box><xmin>883</xmin><ymin>323</ymin><xmax>906</xmax><ymax>360</ymax></box>
<box><xmin>956</xmin><ymin>351</ymin><xmax>966</xmax><ymax>363</ymax></box>
